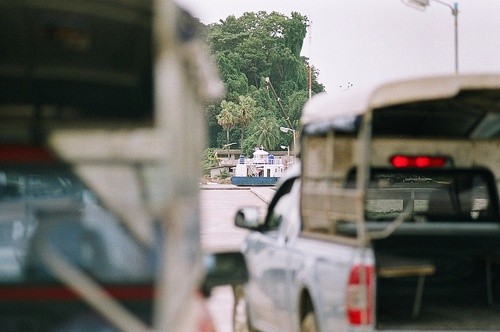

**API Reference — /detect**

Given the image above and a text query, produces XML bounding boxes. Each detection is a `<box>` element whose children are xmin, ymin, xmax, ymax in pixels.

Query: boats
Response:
<box><xmin>230</xmin><ymin>150</ymin><xmax>290</xmax><ymax>186</ymax></box>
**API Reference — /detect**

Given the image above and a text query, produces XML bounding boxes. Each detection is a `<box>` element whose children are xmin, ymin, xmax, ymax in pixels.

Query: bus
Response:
<box><xmin>0</xmin><ymin>0</ymin><xmax>249</xmax><ymax>331</ymax></box>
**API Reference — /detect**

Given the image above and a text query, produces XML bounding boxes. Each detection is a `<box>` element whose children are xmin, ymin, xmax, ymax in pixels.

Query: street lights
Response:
<box><xmin>280</xmin><ymin>145</ymin><xmax>290</xmax><ymax>161</ymax></box>
<box><xmin>280</xmin><ymin>127</ymin><xmax>296</xmax><ymax>161</ymax></box>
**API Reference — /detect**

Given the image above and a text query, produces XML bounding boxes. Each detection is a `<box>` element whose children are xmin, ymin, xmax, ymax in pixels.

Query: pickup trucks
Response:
<box><xmin>232</xmin><ymin>73</ymin><xmax>500</xmax><ymax>332</ymax></box>
